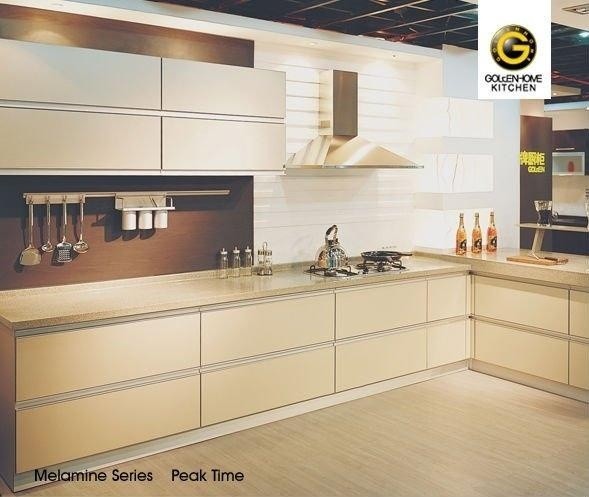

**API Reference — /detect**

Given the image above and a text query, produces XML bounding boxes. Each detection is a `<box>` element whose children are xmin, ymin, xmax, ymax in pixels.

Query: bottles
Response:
<box><xmin>568</xmin><ymin>160</ymin><xmax>574</xmax><ymax>172</ymax></box>
<box><xmin>218</xmin><ymin>246</ymin><xmax>253</xmax><ymax>279</ymax></box>
<box><xmin>257</xmin><ymin>241</ymin><xmax>272</xmax><ymax>276</ymax></box>
<box><xmin>456</xmin><ymin>213</ymin><xmax>467</xmax><ymax>255</ymax></box>
<box><xmin>471</xmin><ymin>212</ymin><xmax>482</xmax><ymax>254</ymax></box>
<box><xmin>485</xmin><ymin>212</ymin><xmax>498</xmax><ymax>252</ymax></box>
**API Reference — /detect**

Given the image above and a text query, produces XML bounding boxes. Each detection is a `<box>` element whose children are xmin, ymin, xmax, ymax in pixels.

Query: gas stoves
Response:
<box><xmin>303</xmin><ymin>260</ymin><xmax>407</xmax><ymax>280</ymax></box>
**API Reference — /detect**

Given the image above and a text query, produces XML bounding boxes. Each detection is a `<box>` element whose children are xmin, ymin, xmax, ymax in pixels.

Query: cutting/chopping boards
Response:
<box><xmin>506</xmin><ymin>253</ymin><xmax>568</xmax><ymax>266</ymax></box>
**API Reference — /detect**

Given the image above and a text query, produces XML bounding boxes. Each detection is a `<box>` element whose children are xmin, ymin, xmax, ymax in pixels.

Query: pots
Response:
<box><xmin>361</xmin><ymin>251</ymin><xmax>412</xmax><ymax>261</ymax></box>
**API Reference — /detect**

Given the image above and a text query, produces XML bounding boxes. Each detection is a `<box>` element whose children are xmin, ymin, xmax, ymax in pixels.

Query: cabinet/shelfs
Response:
<box><xmin>470</xmin><ymin>269</ymin><xmax>589</xmax><ymax>405</ymax></box>
<box><xmin>0</xmin><ymin>269</ymin><xmax>470</xmax><ymax>494</ymax></box>
<box><xmin>0</xmin><ymin>38</ymin><xmax>286</xmax><ymax>177</ymax></box>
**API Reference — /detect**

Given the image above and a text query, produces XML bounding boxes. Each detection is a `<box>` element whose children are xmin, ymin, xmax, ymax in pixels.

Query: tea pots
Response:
<box><xmin>315</xmin><ymin>224</ymin><xmax>348</xmax><ymax>268</ymax></box>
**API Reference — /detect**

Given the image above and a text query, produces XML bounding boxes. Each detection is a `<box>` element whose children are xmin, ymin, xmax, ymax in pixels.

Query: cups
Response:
<box><xmin>534</xmin><ymin>200</ymin><xmax>559</xmax><ymax>227</ymax></box>
<box><xmin>122</xmin><ymin>211</ymin><xmax>136</xmax><ymax>230</ymax></box>
<box><xmin>138</xmin><ymin>211</ymin><xmax>152</xmax><ymax>229</ymax></box>
<box><xmin>154</xmin><ymin>211</ymin><xmax>168</xmax><ymax>229</ymax></box>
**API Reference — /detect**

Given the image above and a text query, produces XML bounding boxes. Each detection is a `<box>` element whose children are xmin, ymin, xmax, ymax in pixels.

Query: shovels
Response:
<box><xmin>56</xmin><ymin>201</ymin><xmax>72</xmax><ymax>262</ymax></box>
<box><xmin>19</xmin><ymin>204</ymin><xmax>41</xmax><ymax>265</ymax></box>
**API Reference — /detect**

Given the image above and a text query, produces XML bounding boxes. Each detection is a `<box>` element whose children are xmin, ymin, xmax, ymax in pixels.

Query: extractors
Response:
<box><xmin>285</xmin><ymin>69</ymin><xmax>424</xmax><ymax>169</ymax></box>
